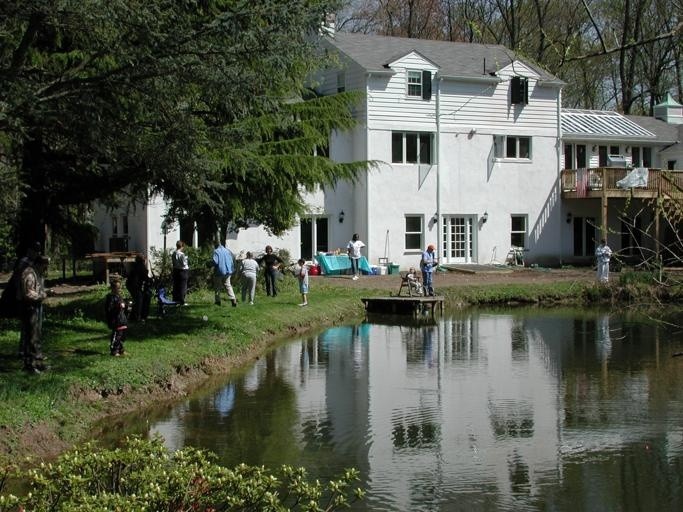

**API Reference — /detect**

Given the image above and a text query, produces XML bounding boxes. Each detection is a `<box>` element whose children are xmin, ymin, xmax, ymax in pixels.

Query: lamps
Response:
<box><xmin>158</xmin><ymin>287</ymin><xmax>183</xmax><ymax>321</ymax></box>
<box><xmin>398</xmin><ymin>275</ymin><xmax>421</xmax><ymax>297</ymax></box>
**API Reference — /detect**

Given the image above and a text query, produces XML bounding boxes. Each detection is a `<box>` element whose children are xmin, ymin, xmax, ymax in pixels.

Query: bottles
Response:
<box><xmin>428</xmin><ymin>245</ymin><xmax>436</xmax><ymax>249</ymax></box>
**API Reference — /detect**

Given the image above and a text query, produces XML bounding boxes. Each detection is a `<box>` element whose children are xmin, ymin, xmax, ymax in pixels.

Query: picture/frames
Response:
<box><xmin>85</xmin><ymin>250</ymin><xmax>144</xmax><ymax>285</ymax></box>
<box><xmin>313</xmin><ymin>255</ymin><xmax>365</xmax><ymax>274</ymax></box>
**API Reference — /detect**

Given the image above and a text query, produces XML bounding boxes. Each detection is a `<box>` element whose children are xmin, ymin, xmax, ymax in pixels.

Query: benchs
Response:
<box><xmin>577</xmin><ymin>167</ymin><xmax>587</xmax><ymax>198</ymax></box>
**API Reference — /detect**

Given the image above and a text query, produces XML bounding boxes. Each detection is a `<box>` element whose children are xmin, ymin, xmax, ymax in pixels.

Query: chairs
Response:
<box><xmin>232</xmin><ymin>299</ymin><xmax>236</xmax><ymax>307</ymax></box>
<box><xmin>352</xmin><ymin>276</ymin><xmax>358</xmax><ymax>280</ymax></box>
<box><xmin>115</xmin><ymin>352</ymin><xmax>131</xmax><ymax>357</ymax></box>
<box><xmin>215</xmin><ymin>303</ymin><xmax>221</xmax><ymax>307</ymax></box>
<box><xmin>248</xmin><ymin>302</ymin><xmax>253</xmax><ymax>305</ymax></box>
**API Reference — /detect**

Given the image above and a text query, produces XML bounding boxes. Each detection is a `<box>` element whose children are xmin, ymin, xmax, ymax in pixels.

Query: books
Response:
<box><xmin>369</xmin><ymin>263</ymin><xmax>400</xmax><ymax>277</ymax></box>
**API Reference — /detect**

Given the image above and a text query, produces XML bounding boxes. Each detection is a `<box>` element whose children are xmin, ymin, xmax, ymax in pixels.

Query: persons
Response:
<box><xmin>406</xmin><ymin>267</ymin><xmax>422</xmax><ymax>294</ymax></box>
<box><xmin>14</xmin><ymin>241</ymin><xmax>47</xmax><ymax>360</ymax></box>
<box><xmin>595</xmin><ymin>239</ymin><xmax>612</xmax><ymax>282</ymax></box>
<box><xmin>20</xmin><ymin>254</ymin><xmax>51</xmax><ymax>375</ymax></box>
<box><xmin>209</xmin><ymin>241</ymin><xmax>237</xmax><ymax>307</ymax></box>
<box><xmin>594</xmin><ymin>308</ymin><xmax>612</xmax><ymax>359</ymax></box>
<box><xmin>240</xmin><ymin>251</ymin><xmax>259</xmax><ymax>305</ymax></box>
<box><xmin>258</xmin><ymin>246</ymin><xmax>283</xmax><ymax>297</ymax></box>
<box><xmin>423</xmin><ymin>327</ymin><xmax>435</xmax><ymax>370</ymax></box>
<box><xmin>420</xmin><ymin>245</ymin><xmax>438</xmax><ymax>296</ymax></box>
<box><xmin>298</xmin><ymin>259</ymin><xmax>310</xmax><ymax>306</ymax></box>
<box><xmin>127</xmin><ymin>255</ymin><xmax>155</xmax><ymax>321</ymax></box>
<box><xmin>172</xmin><ymin>241</ymin><xmax>189</xmax><ymax>306</ymax></box>
<box><xmin>346</xmin><ymin>234</ymin><xmax>366</xmax><ymax>281</ymax></box>
<box><xmin>104</xmin><ymin>279</ymin><xmax>133</xmax><ymax>357</ymax></box>
